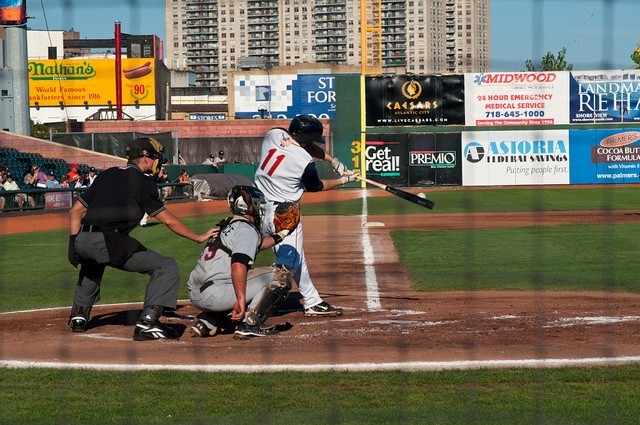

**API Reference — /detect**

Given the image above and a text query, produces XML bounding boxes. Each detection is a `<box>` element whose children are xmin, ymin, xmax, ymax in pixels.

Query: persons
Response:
<box><xmin>46</xmin><ymin>172</ymin><xmax>64</xmax><ymax>188</ymax></box>
<box><xmin>73</xmin><ymin>167</ymin><xmax>97</xmax><ymax>197</ymax></box>
<box><xmin>67</xmin><ymin>163</ymin><xmax>81</xmax><ymax>184</ymax></box>
<box><xmin>187</xmin><ymin>185</ymin><xmax>289</xmax><ymax>340</ymax></box>
<box><xmin>0</xmin><ymin>196</ymin><xmax>6</xmax><ymax>214</ymax></box>
<box><xmin>60</xmin><ymin>174</ymin><xmax>70</xmax><ymax>188</ymax></box>
<box><xmin>177</xmin><ymin>169</ymin><xmax>193</xmax><ymax>199</ymax></box>
<box><xmin>214</xmin><ymin>149</ymin><xmax>229</xmax><ymax>164</ymax></box>
<box><xmin>202</xmin><ymin>154</ymin><xmax>218</xmax><ymax>167</ymax></box>
<box><xmin>21</xmin><ymin>164</ymin><xmax>38</xmax><ymax>188</ymax></box>
<box><xmin>0</xmin><ymin>169</ymin><xmax>6</xmax><ymax>190</ymax></box>
<box><xmin>68</xmin><ymin>137</ymin><xmax>220</xmax><ymax>341</ymax></box>
<box><xmin>31</xmin><ymin>165</ymin><xmax>48</xmax><ymax>188</ymax></box>
<box><xmin>156</xmin><ymin>170</ymin><xmax>176</xmax><ymax>201</ymax></box>
<box><xmin>255</xmin><ymin>115</ymin><xmax>361</xmax><ymax>316</ymax></box>
<box><xmin>178</xmin><ymin>151</ymin><xmax>186</xmax><ymax>165</ymax></box>
<box><xmin>158</xmin><ymin>166</ymin><xmax>166</xmax><ymax>178</ymax></box>
<box><xmin>3</xmin><ymin>172</ymin><xmax>35</xmax><ymax>212</ymax></box>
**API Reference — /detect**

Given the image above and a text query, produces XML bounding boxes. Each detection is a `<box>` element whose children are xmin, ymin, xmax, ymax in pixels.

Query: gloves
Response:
<box><xmin>68</xmin><ymin>234</ymin><xmax>81</xmax><ymax>269</ymax></box>
<box><xmin>330</xmin><ymin>158</ymin><xmax>348</xmax><ymax>176</ymax></box>
<box><xmin>340</xmin><ymin>172</ymin><xmax>361</xmax><ymax>184</ymax></box>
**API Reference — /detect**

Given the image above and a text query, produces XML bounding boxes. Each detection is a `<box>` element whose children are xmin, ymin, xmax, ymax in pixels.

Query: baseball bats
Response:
<box><xmin>356</xmin><ymin>172</ymin><xmax>438</xmax><ymax>212</ymax></box>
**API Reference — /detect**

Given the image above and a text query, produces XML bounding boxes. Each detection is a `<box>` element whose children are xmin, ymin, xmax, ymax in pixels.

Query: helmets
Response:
<box><xmin>126</xmin><ymin>137</ymin><xmax>168</xmax><ymax>175</ymax></box>
<box><xmin>289</xmin><ymin>115</ymin><xmax>326</xmax><ymax>146</ymax></box>
<box><xmin>228</xmin><ymin>185</ymin><xmax>267</xmax><ymax>227</ymax></box>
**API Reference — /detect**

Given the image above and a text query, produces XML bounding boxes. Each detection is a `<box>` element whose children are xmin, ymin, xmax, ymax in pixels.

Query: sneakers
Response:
<box><xmin>133</xmin><ymin>319</ymin><xmax>178</xmax><ymax>341</ymax></box>
<box><xmin>190</xmin><ymin>320</ymin><xmax>211</xmax><ymax>337</ymax></box>
<box><xmin>70</xmin><ymin>317</ymin><xmax>87</xmax><ymax>331</ymax></box>
<box><xmin>304</xmin><ymin>303</ymin><xmax>344</xmax><ymax>317</ymax></box>
<box><xmin>233</xmin><ymin>322</ymin><xmax>280</xmax><ymax>340</ymax></box>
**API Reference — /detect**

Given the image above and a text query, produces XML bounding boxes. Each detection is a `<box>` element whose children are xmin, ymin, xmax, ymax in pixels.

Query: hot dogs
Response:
<box><xmin>122</xmin><ymin>60</ymin><xmax>153</xmax><ymax>80</ymax></box>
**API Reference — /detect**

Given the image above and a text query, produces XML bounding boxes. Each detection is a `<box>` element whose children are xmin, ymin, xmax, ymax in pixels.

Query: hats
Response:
<box><xmin>48</xmin><ymin>171</ymin><xmax>54</xmax><ymax>176</ymax></box>
<box><xmin>218</xmin><ymin>150</ymin><xmax>224</xmax><ymax>155</ymax></box>
<box><xmin>6</xmin><ymin>172</ymin><xmax>13</xmax><ymax>177</ymax></box>
<box><xmin>70</xmin><ymin>164</ymin><xmax>79</xmax><ymax>169</ymax></box>
<box><xmin>90</xmin><ymin>167</ymin><xmax>95</xmax><ymax>172</ymax></box>
<box><xmin>209</xmin><ymin>154</ymin><xmax>214</xmax><ymax>158</ymax></box>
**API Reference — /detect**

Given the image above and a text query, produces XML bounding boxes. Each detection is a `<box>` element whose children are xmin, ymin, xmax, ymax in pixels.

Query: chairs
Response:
<box><xmin>0</xmin><ymin>146</ymin><xmax>104</xmax><ymax>204</ymax></box>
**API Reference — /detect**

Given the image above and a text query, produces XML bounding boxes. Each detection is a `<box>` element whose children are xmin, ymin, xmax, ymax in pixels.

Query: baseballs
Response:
<box><xmin>417</xmin><ymin>193</ymin><xmax>426</xmax><ymax>200</ymax></box>
